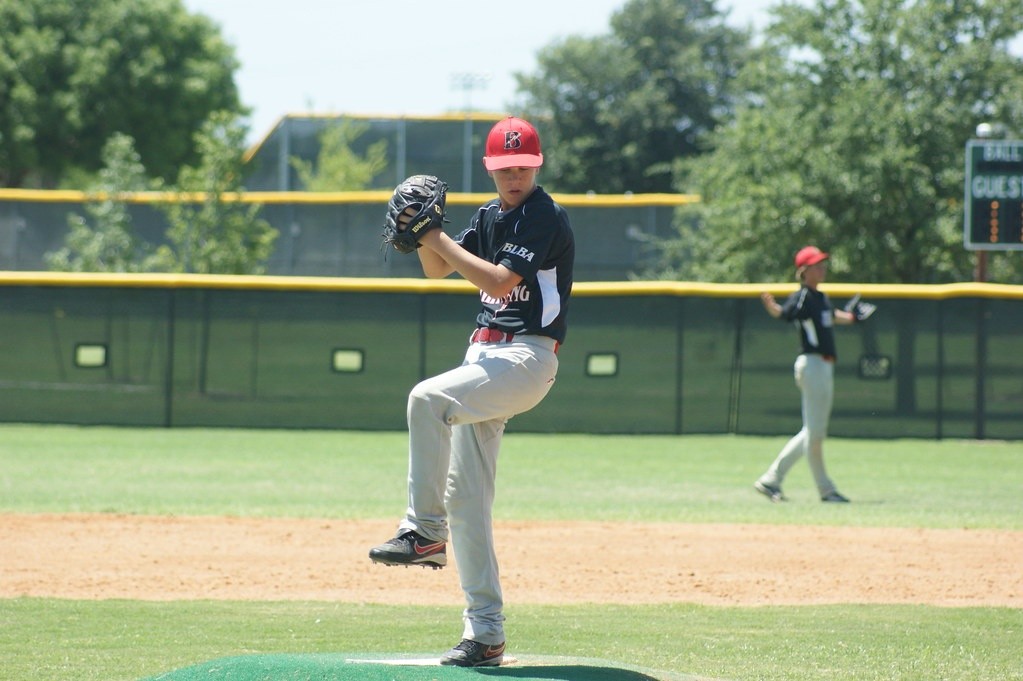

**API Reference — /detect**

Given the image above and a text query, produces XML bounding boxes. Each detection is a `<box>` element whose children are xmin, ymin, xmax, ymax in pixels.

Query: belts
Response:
<box><xmin>473</xmin><ymin>328</ymin><xmax>562</xmax><ymax>354</ymax></box>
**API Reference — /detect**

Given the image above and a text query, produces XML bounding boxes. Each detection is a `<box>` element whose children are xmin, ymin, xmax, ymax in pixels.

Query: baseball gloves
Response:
<box><xmin>384</xmin><ymin>175</ymin><xmax>449</xmax><ymax>254</ymax></box>
<box><xmin>849</xmin><ymin>294</ymin><xmax>878</xmax><ymax>324</ymax></box>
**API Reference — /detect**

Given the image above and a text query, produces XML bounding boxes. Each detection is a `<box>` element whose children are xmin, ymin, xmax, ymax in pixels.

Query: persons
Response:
<box><xmin>755</xmin><ymin>246</ymin><xmax>878</xmax><ymax>502</ymax></box>
<box><xmin>368</xmin><ymin>113</ymin><xmax>575</xmax><ymax>669</ymax></box>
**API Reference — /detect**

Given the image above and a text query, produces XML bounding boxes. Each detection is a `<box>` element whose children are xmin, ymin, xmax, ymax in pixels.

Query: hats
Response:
<box><xmin>795</xmin><ymin>245</ymin><xmax>828</xmax><ymax>268</ymax></box>
<box><xmin>484</xmin><ymin>115</ymin><xmax>544</xmax><ymax>172</ymax></box>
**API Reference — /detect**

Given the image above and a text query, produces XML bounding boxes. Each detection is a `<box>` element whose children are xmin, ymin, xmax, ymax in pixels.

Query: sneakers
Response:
<box><xmin>439</xmin><ymin>639</ymin><xmax>506</xmax><ymax>666</ymax></box>
<box><xmin>754</xmin><ymin>479</ymin><xmax>790</xmax><ymax>504</ymax></box>
<box><xmin>822</xmin><ymin>490</ymin><xmax>851</xmax><ymax>503</ymax></box>
<box><xmin>368</xmin><ymin>531</ymin><xmax>447</xmax><ymax>570</ymax></box>
